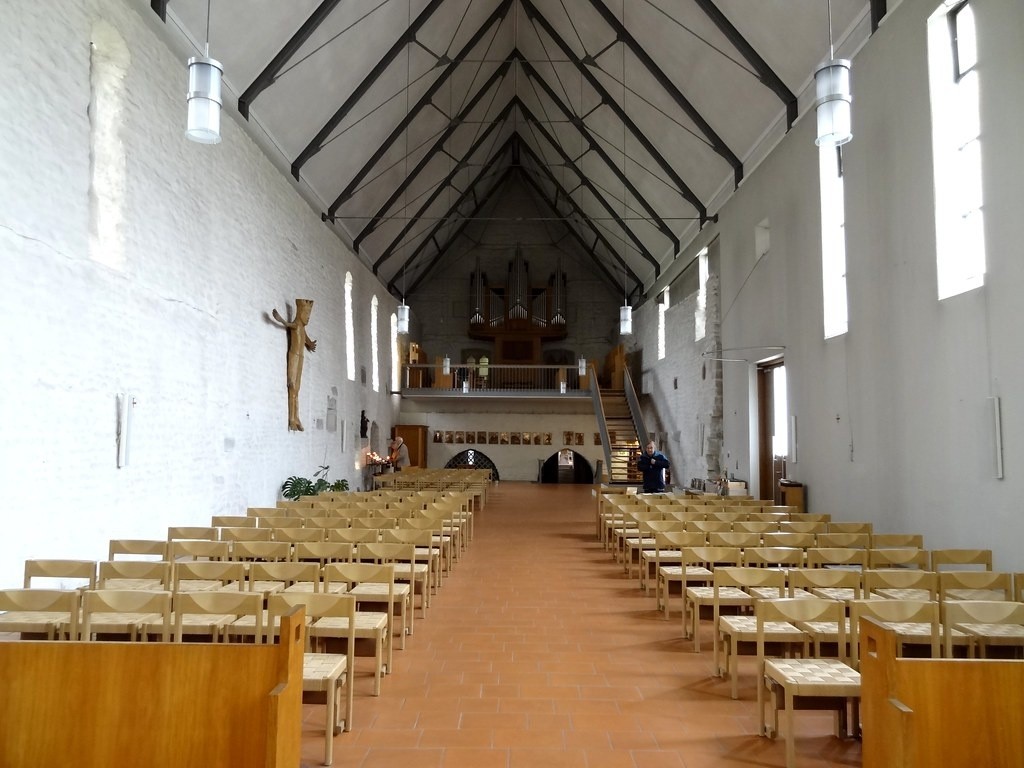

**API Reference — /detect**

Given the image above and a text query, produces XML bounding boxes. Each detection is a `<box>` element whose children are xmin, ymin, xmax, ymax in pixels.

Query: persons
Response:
<box><xmin>273</xmin><ymin>297</ymin><xmax>317</xmax><ymax>431</ymax></box>
<box><xmin>390</xmin><ymin>442</ymin><xmax>401</xmax><ymax>473</ymax></box>
<box><xmin>392</xmin><ymin>436</ymin><xmax>410</xmax><ymax>469</ymax></box>
<box><xmin>637</xmin><ymin>441</ymin><xmax>670</xmax><ymax>493</ymax></box>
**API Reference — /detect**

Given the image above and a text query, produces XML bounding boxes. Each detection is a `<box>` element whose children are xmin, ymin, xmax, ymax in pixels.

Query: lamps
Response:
<box><xmin>185</xmin><ymin>0</ymin><xmax>224</xmax><ymax>144</ymax></box>
<box><xmin>341</xmin><ymin>419</ymin><xmax>346</xmax><ymax>453</ymax></box>
<box><xmin>397</xmin><ymin>0</ymin><xmax>411</xmax><ymax>333</ymax></box>
<box><xmin>619</xmin><ymin>0</ymin><xmax>633</xmax><ymax>334</ymax></box>
<box><xmin>579</xmin><ymin>62</ymin><xmax>586</xmax><ymax>375</ymax></box>
<box><xmin>463</xmin><ymin>163</ymin><xmax>471</xmax><ymax>393</ymax></box>
<box><xmin>659</xmin><ymin>432</ymin><xmax>667</xmax><ymax>440</ymax></box>
<box><xmin>988</xmin><ymin>396</ymin><xmax>1002</xmax><ymax>479</ymax></box>
<box><xmin>117</xmin><ymin>394</ymin><xmax>128</xmax><ymax>467</ymax></box>
<box><xmin>697</xmin><ymin>423</ymin><xmax>703</xmax><ymax>457</ymax></box>
<box><xmin>560</xmin><ymin>166</ymin><xmax>567</xmax><ymax>393</ymax></box>
<box><xmin>791</xmin><ymin>416</ymin><xmax>796</xmax><ymax>462</ymax></box>
<box><xmin>443</xmin><ymin>63</ymin><xmax>451</xmax><ymax>375</ymax></box>
<box><xmin>670</xmin><ymin>431</ymin><xmax>680</xmax><ymax>441</ymax></box>
<box><xmin>812</xmin><ymin>0</ymin><xmax>854</xmax><ymax>148</ymax></box>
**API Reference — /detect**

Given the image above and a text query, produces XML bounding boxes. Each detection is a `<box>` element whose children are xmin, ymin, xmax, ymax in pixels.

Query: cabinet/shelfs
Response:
<box><xmin>409</xmin><ymin>341</ymin><xmax>427</xmax><ymax>388</ymax></box>
<box><xmin>603</xmin><ymin>343</ymin><xmax>624</xmax><ymax>389</ymax></box>
<box><xmin>395</xmin><ymin>424</ymin><xmax>428</xmax><ymax>469</ymax></box>
<box><xmin>778</xmin><ymin>486</ymin><xmax>804</xmax><ymax>513</ymax></box>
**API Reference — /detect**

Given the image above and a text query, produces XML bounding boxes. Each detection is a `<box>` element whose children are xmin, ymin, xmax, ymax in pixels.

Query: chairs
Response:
<box><xmin>0</xmin><ymin>465</ymin><xmax>493</xmax><ymax>768</ymax></box>
<box><xmin>594</xmin><ymin>482</ymin><xmax>1023</xmax><ymax>768</ymax></box>
